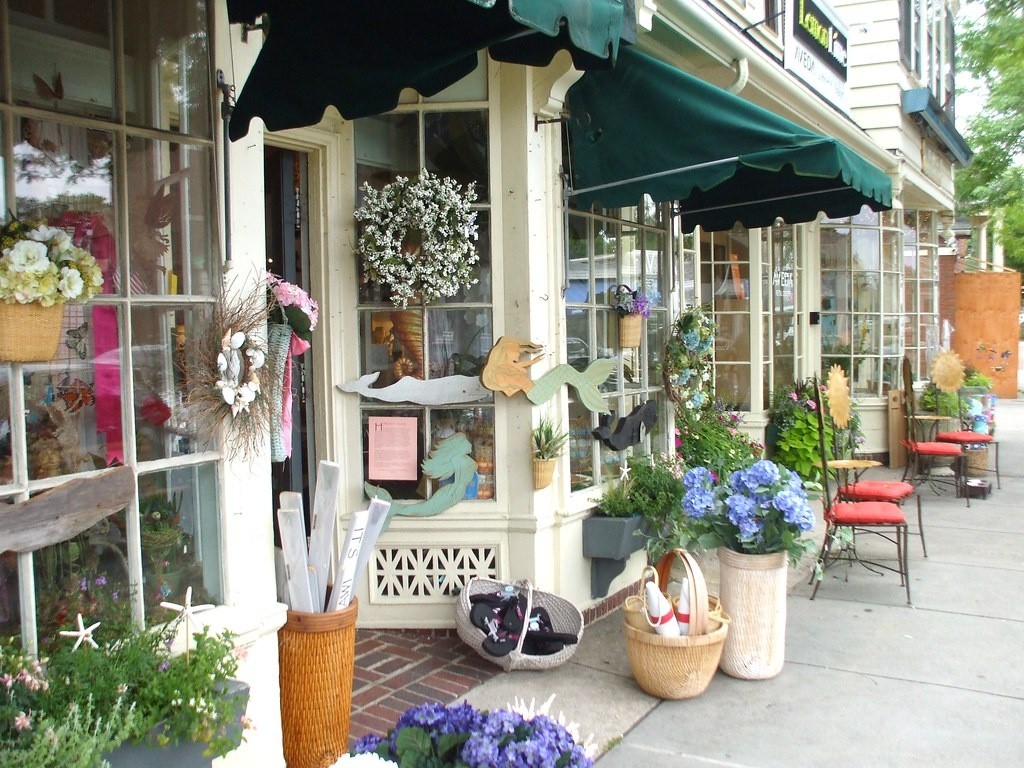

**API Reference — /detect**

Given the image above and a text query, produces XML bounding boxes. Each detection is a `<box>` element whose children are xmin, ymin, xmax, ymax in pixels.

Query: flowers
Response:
<box><xmin>327</xmin><ymin>699</ymin><xmax>596</xmax><ymax>768</ymax></box>
<box><xmin>351</xmin><ymin>166</ymin><xmax>480</xmax><ymax>305</ymax></box>
<box><xmin>0</xmin><ymin>207</ymin><xmax>105</xmax><ymax>308</ymax></box>
<box><xmin>611</xmin><ymin>290</ymin><xmax>651</xmax><ymax>318</ymax></box>
<box><xmin>582</xmin><ymin>304</ymin><xmax>865</xmax><ymax>582</ymax></box>
<box><xmin>266</xmin><ymin>269</ymin><xmax>319</xmax><ymax>331</ymax></box>
<box><xmin>1</xmin><ymin>489</ymin><xmax>248</xmax><ymax>768</ymax></box>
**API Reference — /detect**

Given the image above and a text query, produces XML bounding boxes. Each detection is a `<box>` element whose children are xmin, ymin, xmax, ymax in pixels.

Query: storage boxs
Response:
<box><xmin>959</xmin><ymin>480</ymin><xmax>993</xmax><ymax>499</ymax></box>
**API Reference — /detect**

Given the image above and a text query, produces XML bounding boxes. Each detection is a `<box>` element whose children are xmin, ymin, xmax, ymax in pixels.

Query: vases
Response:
<box><xmin>146</xmin><ymin>567</ymin><xmax>183</xmax><ymax>597</ymax></box>
<box><xmin>102</xmin><ymin>678</ymin><xmax>250</xmax><ymax>768</ymax></box>
<box><xmin>583</xmin><ymin>514</ymin><xmax>656</xmax><ymax>599</ymax></box>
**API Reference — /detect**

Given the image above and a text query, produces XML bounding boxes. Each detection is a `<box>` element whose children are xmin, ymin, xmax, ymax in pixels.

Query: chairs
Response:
<box><xmin>809</xmin><ymin>349</ymin><xmax>1000</xmax><ymax>604</ymax></box>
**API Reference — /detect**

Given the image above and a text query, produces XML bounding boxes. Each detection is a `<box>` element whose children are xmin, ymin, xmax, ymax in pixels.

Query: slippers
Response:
<box><xmin>469</xmin><ymin>590</ymin><xmax>578</xmax><ymax>657</ymax></box>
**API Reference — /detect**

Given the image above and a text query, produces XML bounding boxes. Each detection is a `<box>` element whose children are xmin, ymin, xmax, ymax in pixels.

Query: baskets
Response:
<box><xmin>531</xmin><ymin>428</ymin><xmax>558</xmax><ymax>490</ymax></box>
<box><xmin>455</xmin><ymin>577</ymin><xmax>584</xmax><ymax>671</ymax></box>
<box><xmin>622</xmin><ymin>565</ymin><xmax>673</xmax><ymax>634</ymax></box>
<box><xmin>620</xmin><ymin>547</ymin><xmax>730</xmax><ymax>699</ymax></box>
<box><xmin>673</xmin><ymin>588</ymin><xmax>732</xmax><ymax>634</ymax></box>
<box><xmin>616</xmin><ymin>284</ymin><xmax>642</xmax><ymax>347</ymax></box>
<box><xmin>1</xmin><ymin>295</ymin><xmax>64</xmax><ymax>361</ymax></box>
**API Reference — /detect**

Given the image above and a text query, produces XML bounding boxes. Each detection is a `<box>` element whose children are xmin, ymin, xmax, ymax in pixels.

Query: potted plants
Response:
<box><xmin>531</xmin><ymin>411</ymin><xmax>569</xmax><ymax>490</ymax></box>
